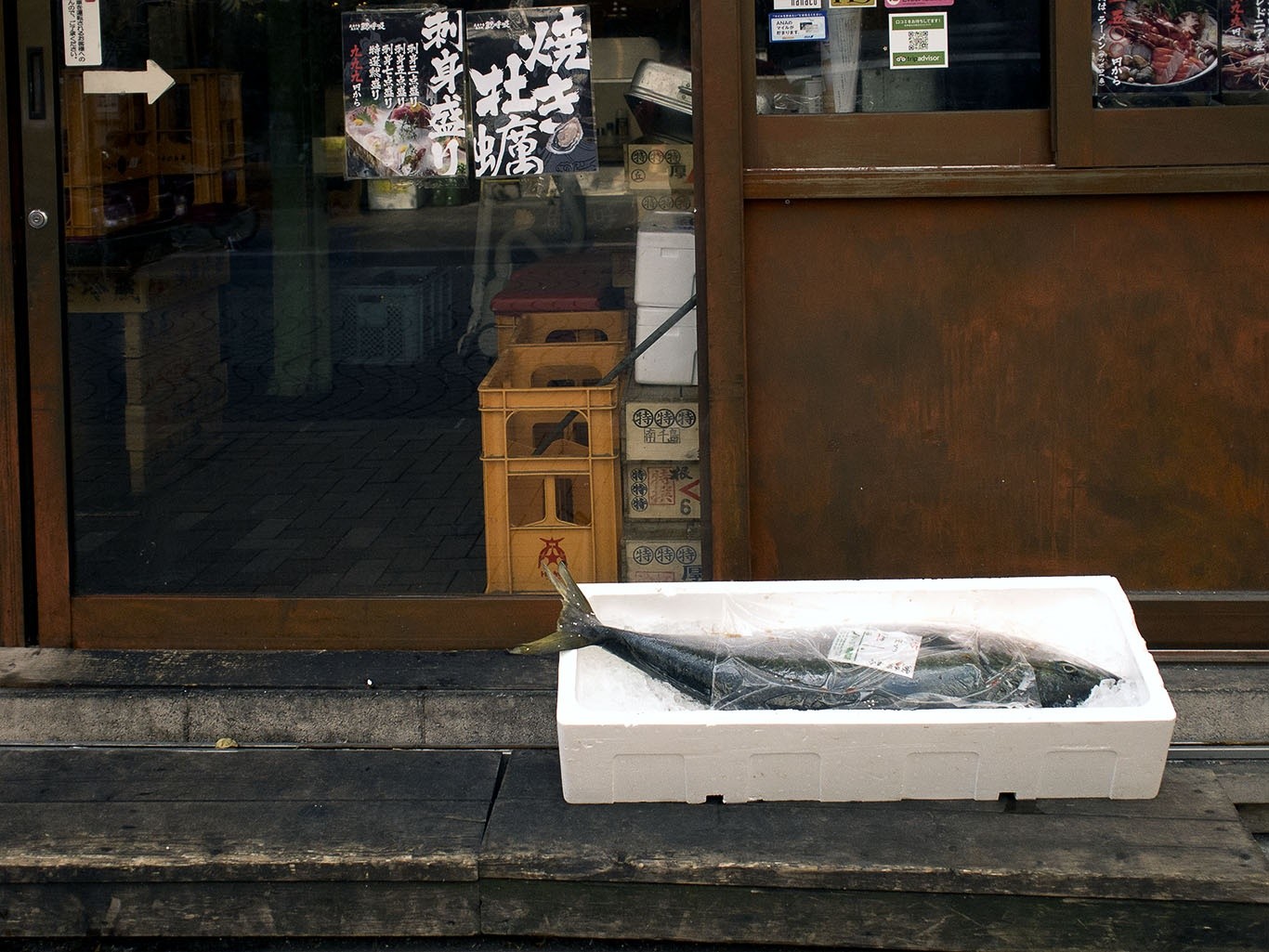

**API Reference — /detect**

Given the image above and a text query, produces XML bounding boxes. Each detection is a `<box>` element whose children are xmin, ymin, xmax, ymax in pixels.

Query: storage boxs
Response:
<box><xmin>160</xmin><ymin>68</ymin><xmax>249</xmax><ymax>207</ymax></box>
<box><xmin>619</xmin><ymin>523</ymin><xmax>702</xmax><ymax>582</ymax></box>
<box><xmin>634</xmin><ymin>212</ymin><xmax>696</xmax><ymax>308</ymax></box>
<box><xmin>559</xmin><ymin>575</ymin><xmax>1176</xmax><ymax>805</ymax></box>
<box><xmin>624</xmin><ymin>462</ymin><xmax>700</xmax><ymax>520</ymax></box>
<box><xmin>619</xmin><ymin>370</ymin><xmax>699</xmax><ymax>461</ymax></box>
<box><xmin>229</xmin><ymin>266</ymin><xmax>452</xmax><ymax>366</ymax></box>
<box><xmin>368</xmin><ymin>178</ymin><xmax>426</xmax><ymax>210</ymax></box>
<box><xmin>312</xmin><ymin>137</ymin><xmax>343</xmax><ymax>173</ymax></box>
<box><xmin>477</xmin><ymin>312</ymin><xmax>621</xmax><ymax>593</ymax></box>
<box><xmin>635</xmin><ymin>307</ymin><xmax>698</xmax><ymax>386</ymax></box>
<box><xmin>54</xmin><ymin>66</ymin><xmax>159</xmax><ymax>239</ymax></box>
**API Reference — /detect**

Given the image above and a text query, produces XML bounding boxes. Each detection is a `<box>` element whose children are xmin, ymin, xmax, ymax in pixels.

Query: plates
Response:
<box><xmin>1091</xmin><ymin>15</ymin><xmax>1217</xmax><ymax>87</ymax></box>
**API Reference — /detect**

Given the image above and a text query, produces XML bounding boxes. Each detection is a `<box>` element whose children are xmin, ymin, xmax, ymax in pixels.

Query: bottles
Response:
<box><xmin>616</xmin><ymin>109</ymin><xmax>629</xmax><ymax>139</ymax></box>
<box><xmin>606</xmin><ymin>122</ymin><xmax>614</xmax><ymax>138</ymax></box>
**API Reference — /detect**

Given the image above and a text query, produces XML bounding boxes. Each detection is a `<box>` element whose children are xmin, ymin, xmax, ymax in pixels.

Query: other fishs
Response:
<box><xmin>508</xmin><ymin>556</ymin><xmax>1128</xmax><ymax>710</ymax></box>
